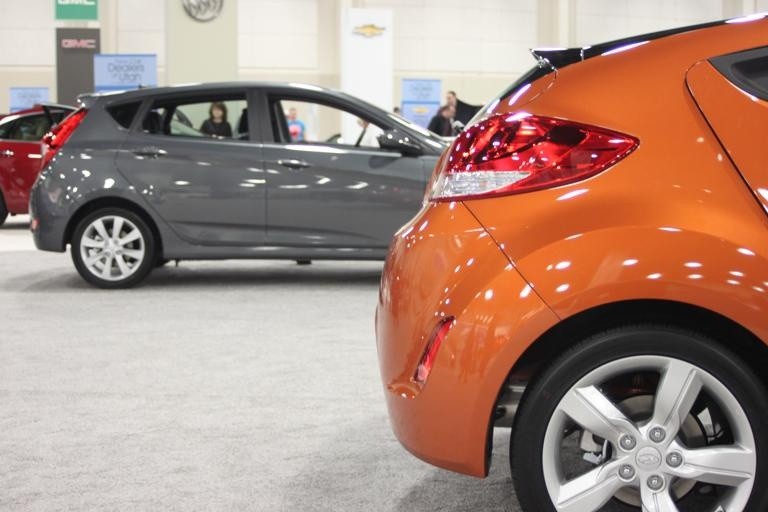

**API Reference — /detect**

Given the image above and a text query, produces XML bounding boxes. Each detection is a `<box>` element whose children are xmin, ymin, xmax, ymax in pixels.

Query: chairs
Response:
<box><xmin>143</xmin><ymin>108</ymin><xmax>249</xmax><ymax>139</ymax></box>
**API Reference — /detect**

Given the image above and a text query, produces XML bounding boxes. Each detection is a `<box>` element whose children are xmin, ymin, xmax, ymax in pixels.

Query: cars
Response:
<box><xmin>368</xmin><ymin>12</ymin><xmax>768</xmax><ymax>510</ymax></box>
<box><xmin>0</xmin><ymin>102</ymin><xmax>76</xmax><ymax>228</ymax></box>
<box><xmin>25</xmin><ymin>80</ymin><xmax>457</xmax><ymax>286</ymax></box>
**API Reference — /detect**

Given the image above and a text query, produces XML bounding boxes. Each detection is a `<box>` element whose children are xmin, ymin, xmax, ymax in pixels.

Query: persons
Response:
<box><xmin>427</xmin><ymin>104</ymin><xmax>456</xmax><ymax>136</ymax></box>
<box><xmin>446</xmin><ymin>89</ymin><xmax>457</xmax><ymax>107</ymax></box>
<box><xmin>287</xmin><ymin>107</ymin><xmax>307</xmax><ymax>141</ymax></box>
<box><xmin>199</xmin><ymin>102</ymin><xmax>231</xmax><ymax>137</ymax></box>
